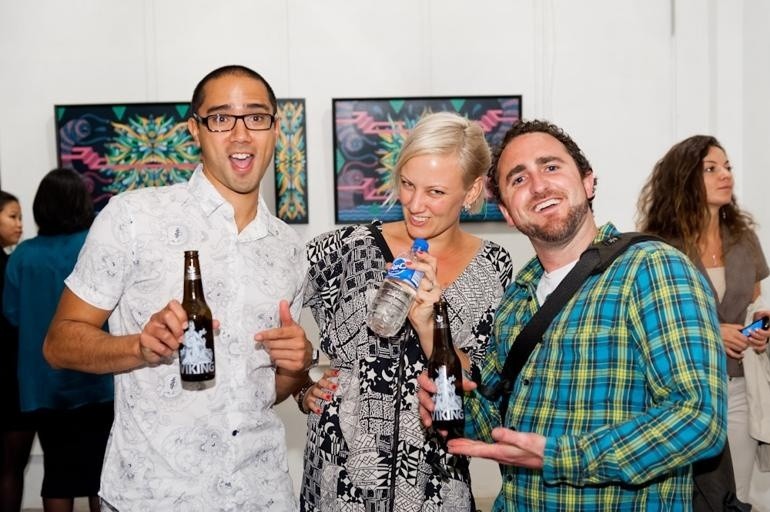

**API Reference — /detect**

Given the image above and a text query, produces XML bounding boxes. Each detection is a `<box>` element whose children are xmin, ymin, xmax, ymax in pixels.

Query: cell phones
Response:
<box><xmin>739</xmin><ymin>315</ymin><xmax>770</xmax><ymax>338</ymax></box>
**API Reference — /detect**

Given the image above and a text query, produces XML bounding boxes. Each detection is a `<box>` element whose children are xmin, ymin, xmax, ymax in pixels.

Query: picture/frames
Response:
<box><xmin>331</xmin><ymin>95</ymin><xmax>523</xmax><ymax>225</ymax></box>
<box><xmin>53</xmin><ymin>98</ymin><xmax>309</xmax><ymax>225</ymax></box>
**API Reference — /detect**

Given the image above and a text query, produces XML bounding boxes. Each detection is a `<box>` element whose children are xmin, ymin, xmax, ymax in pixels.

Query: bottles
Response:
<box><xmin>176</xmin><ymin>250</ymin><xmax>216</xmax><ymax>383</ymax></box>
<box><xmin>428</xmin><ymin>302</ymin><xmax>465</xmax><ymax>433</ymax></box>
<box><xmin>364</xmin><ymin>238</ymin><xmax>429</xmax><ymax>339</ymax></box>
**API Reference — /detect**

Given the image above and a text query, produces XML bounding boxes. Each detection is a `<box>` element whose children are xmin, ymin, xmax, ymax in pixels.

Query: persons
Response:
<box><xmin>294</xmin><ymin>111</ymin><xmax>514</xmax><ymax>511</ymax></box>
<box><xmin>0</xmin><ymin>188</ymin><xmax>43</xmax><ymax>510</ymax></box>
<box><xmin>419</xmin><ymin>119</ymin><xmax>726</xmax><ymax>511</ymax></box>
<box><xmin>637</xmin><ymin>137</ymin><xmax>768</xmax><ymax>512</ymax></box>
<box><xmin>43</xmin><ymin>65</ymin><xmax>313</xmax><ymax>512</ymax></box>
<box><xmin>5</xmin><ymin>168</ymin><xmax>112</xmax><ymax>512</ymax></box>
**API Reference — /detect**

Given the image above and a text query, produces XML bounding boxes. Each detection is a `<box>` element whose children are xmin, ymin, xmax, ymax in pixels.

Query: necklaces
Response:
<box><xmin>698</xmin><ymin>242</ymin><xmax>722</xmax><ymax>269</ymax></box>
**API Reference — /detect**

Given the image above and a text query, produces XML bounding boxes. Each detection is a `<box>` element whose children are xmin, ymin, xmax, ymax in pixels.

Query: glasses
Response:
<box><xmin>191</xmin><ymin>113</ymin><xmax>276</xmax><ymax>132</ymax></box>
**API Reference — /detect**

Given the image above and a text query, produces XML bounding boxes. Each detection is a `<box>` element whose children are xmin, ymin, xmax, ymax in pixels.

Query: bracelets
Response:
<box><xmin>299</xmin><ymin>383</ymin><xmax>311</xmax><ymax>415</ymax></box>
